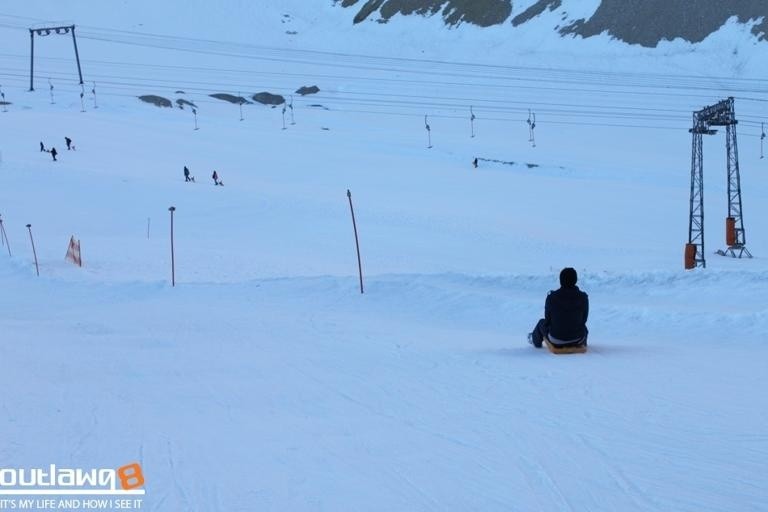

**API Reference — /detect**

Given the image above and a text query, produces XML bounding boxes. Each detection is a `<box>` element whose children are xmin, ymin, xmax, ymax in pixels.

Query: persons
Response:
<box><xmin>50</xmin><ymin>147</ymin><xmax>58</xmax><ymax>160</ymax></box>
<box><xmin>184</xmin><ymin>166</ymin><xmax>190</xmax><ymax>182</ymax></box>
<box><xmin>526</xmin><ymin>267</ymin><xmax>589</xmax><ymax>351</ymax></box>
<box><xmin>65</xmin><ymin>137</ymin><xmax>72</xmax><ymax>150</ymax></box>
<box><xmin>212</xmin><ymin>171</ymin><xmax>219</xmax><ymax>185</ymax></box>
<box><xmin>40</xmin><ymin>141</ymin><xmax>45</xmax><ymax>152</ymax></box>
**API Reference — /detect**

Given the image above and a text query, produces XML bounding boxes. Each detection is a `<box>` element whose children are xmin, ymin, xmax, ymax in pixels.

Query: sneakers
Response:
<box><xmin>528</xmin><ymin>333</ymin><xmax>535</xmax><ymax>346</ymax></box>
